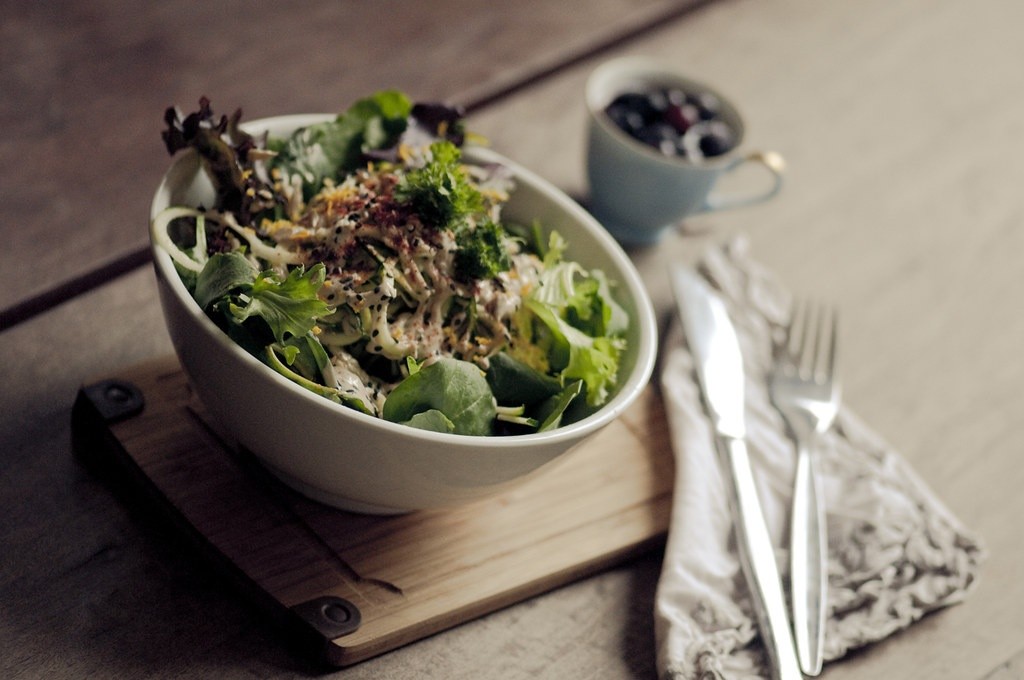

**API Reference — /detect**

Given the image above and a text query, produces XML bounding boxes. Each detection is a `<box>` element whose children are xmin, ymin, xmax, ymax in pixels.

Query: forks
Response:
<box><xmin>768</xmin><ymin>292</ymin><xmax>842</xmax><ymax>676</ymax></box>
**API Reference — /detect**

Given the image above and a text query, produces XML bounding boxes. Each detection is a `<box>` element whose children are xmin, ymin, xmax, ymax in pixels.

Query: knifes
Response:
<box><xmin>666</xmin><ymin>254</ymin><xmax>801</xmax><ymax>679</ymax></box>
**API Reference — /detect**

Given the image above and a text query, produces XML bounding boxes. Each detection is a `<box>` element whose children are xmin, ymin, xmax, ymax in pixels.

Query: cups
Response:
<box><xmin>583</xmin><ymin>58</ymin><xmax>786</xmax><ymax>247</ymax></box>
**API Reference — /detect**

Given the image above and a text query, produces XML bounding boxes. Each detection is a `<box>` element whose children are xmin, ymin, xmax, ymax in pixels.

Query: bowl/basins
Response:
<box><xmin>147</xmin><ymin>113</ymin><xmax>660</xmax><ymax>517</ymax></box>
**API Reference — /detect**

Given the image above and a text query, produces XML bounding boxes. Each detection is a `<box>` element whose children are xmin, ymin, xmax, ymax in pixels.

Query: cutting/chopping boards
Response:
<box><xmin>74</xmin><ymin>349</ymin><xmax>672</xmax><ymax>667</ymax></box>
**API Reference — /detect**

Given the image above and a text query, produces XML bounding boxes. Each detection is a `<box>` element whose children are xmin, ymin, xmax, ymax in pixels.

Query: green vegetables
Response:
<box><xmin>156</xmin><ymin>83</ymin><xmax>630</xmax><ymax>435</ymax></box>
